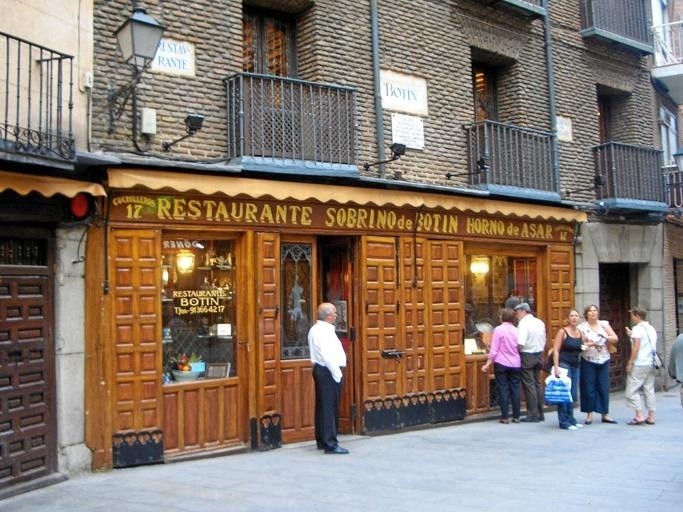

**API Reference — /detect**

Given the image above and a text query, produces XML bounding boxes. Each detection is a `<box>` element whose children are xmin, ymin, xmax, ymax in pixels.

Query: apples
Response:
<box><xmin>173</xmin><ymin>363</ymin><xmax>184</xmax><ymax>371</ymax></box>
<box><xmin>181</xmin><ymin>353</ymin><xmax>188</xmax><ymax>362</ymax></box>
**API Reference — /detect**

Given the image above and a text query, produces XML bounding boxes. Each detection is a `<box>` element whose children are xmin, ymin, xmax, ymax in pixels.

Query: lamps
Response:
<box><xmin>446</xmin><ymin>158</ymin><xmax>490</xmax><ymax>180</ymax></box>
<box><xmin>107</xmin><ymin>0</ymin><xmax>168</xmax><ymax>153</ymax></box>
<box><xmin>565</xmin><ymin>176</ymin><xmax>604</xmax><ymax>197</ymax></box>
<box><xmin>161</xmin><ymin>249</ymin><xmax>196</xmax><ymax>286</ymax></box>
<box><xmin>162</xmin><ymin>114</ymin><xmax>204</xmax><ymax>150</ymax></box>
<box><xmin>363</xmin><ymin>143</ymin><xmax>406</xmax><ymax>172</ymax></box>
<box><xmin>470</xmin><ymin>254</ymin><xmax>490</xmax><ymax>274</ymax></box>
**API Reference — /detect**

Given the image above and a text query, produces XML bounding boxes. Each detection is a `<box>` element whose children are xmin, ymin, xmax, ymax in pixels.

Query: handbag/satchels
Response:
<box><xmin>542</xmin><ymin>350</ymin><xmax>554</xmax><ymax>374</ymax></box>
<box><xmin>653</xmin><ymin>352</ymin><xmax>665</xmax><ymax>369</ymax></box>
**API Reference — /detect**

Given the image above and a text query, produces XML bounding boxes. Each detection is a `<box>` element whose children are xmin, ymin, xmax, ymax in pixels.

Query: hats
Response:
<box><xmin>514</xmin><ymin>302</ymin><xmax>531</xmax><ymax>312</ymax></box>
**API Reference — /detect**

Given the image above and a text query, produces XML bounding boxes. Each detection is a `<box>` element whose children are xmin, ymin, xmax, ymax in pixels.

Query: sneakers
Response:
<box><xmin>499</xmin><ymin>419</ymin><xmax>508</xmax><ymax>423</ymax></box>
<box><xmin>511</xmin><ymin>418</ymin><xmax>519</xmax><ymax>423</ymax></box>
<box><xmin>568</xmin><ymin>424</ymin><xmax>583</xmax><ymax>430</ymax></box>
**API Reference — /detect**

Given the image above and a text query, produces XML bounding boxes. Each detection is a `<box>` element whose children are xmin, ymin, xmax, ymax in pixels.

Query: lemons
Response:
<box><xmin>182</xmin><ymin>365</ymin><xmax>190</xmax><ymax>371</ymax></box>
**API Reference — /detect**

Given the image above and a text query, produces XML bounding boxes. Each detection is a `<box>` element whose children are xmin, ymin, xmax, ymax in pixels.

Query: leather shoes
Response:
<box><xmin>601</xmin><ymin>418</ymin><xmax>618</xmax><ymax>423</ymax></box>
<box><xmin>520</xmin><ymin>418</ymin><xmax>545</xmax><ymax>422</ymax></box>
<box><xmin>585</xmin><ymin>420</ymin><xmax>592</xmax><ymax>425</ymax></box>
<box><xmin>318</xmin><ymin>441</ymin><xmax>349</xmax><ymax>454</ymax></box>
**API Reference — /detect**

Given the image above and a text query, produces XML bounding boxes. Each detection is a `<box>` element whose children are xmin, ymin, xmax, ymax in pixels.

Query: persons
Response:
<box><xmin>666</xmin><ymin>331</ymin><xmax>683</xmax><ymax>412</ymax></box>
<box><xmin>575</xmin><ymin>303</ymin><xmax>620</xmax><ymax>425</ymax></box>
<box><xmin>305</xmin><ymin>301</ymin><xmax>352</xmax><ymax>455</ymax></box>
<box><xmin>549</xmin><ymin>309</ymin><xmax>590</xmax><ymax>430</ymax></box>
<box><xmin>512</xmin><ymin>301</ymin><xmax>547</xmax><ymax>423</ymax></box>
<box><xmin>480</xmin><ymin>307</ymin><xmax>521</xmax><ymax>424</ymax></box>
<box><xmin>623</xmin><ymin>307</ymin><xmax>658</xmax><ymax>425</ymax></box>
<box><xmin>464</xmin><ymin>302</ymin><xmax>483</xmax><ymax>338</ymax></box>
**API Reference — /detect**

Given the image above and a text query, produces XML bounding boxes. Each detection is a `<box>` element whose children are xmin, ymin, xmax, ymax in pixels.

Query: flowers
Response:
<box><xmin>170</xmin><ymin>352</ymin><xmax>204</xmax><ymax>371</ymax></box>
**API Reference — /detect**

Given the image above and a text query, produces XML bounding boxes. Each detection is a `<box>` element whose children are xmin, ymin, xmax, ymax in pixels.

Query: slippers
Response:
<box><xmin>626</xmin><ymin>418</ymin><xmax>644</xmax><ymax>425</ymax></box>
<box><xmin>645</xmin><ymin>419</ymin><xmax>655</xmax><ymax>424</ymax></box>
